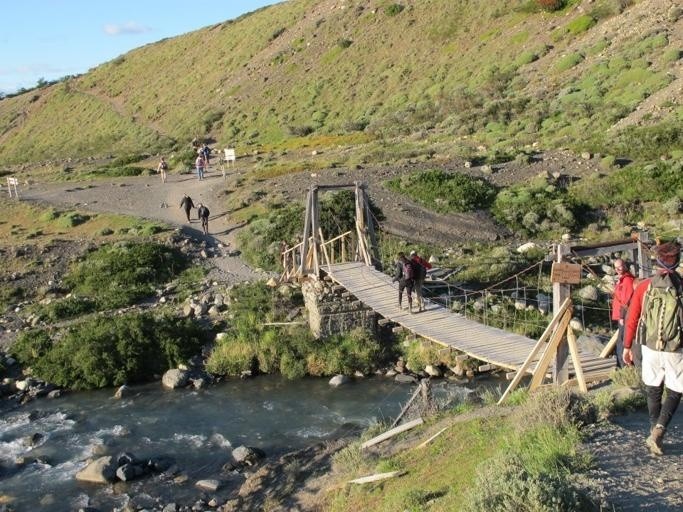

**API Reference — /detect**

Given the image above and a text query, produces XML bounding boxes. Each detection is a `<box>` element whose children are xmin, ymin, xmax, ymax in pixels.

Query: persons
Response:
<box><xmin>157</xmin><ymin>156</ymin><xmax>167</xmax><ymax>183</ymax></box>
<box><xmin>611</xmin><ymin>242</ymin><xmax>683</xmax><ymax>455</ymax></box>
<box><xmin>195</xmin><ymin>144</ymin><xmax>210</xmax><ymax>181</ymax></box>
<box><xmin>198</xmin><ymin>203</ymin><xmax>210</xmax><ymax>236</ymax></box>
<box><xmin>178</xmin><ymin>193</ymin><xmax>194</xmax><ymax>223</ymax></box>
<box><xmin>392</xmin><ymin>251</ymin><xmax>432</xmax><ymax>313</ymax></box>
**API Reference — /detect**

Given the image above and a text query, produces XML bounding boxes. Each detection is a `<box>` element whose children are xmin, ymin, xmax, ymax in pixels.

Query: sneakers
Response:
<box><xmin>399</xmin><ymin>306</ymin><xmax>425</xmax><ymax>314</ymax></box>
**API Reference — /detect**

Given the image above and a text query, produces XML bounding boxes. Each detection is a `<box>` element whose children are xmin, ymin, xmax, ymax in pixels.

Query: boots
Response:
<box><xmin>646</xmin><ymin>424</ymin><xmax>665</xmax><ymax>455</ymax></box>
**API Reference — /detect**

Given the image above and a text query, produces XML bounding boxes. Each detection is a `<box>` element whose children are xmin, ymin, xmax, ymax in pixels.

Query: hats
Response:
<box><xmin>655</xmin><ymin>242</ymin><xmax>680</xmax><ymax>269</ymax></box>
<box><xmin>410</xmin><ymin>251</ymin><xmax>415</xmax><ymax>256</ymax></box>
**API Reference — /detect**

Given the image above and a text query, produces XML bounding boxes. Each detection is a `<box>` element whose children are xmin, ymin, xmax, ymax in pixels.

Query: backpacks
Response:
<box><xmin>636</xmin><ymin>272</ymin><xmax>683</xmax><ymax>352</ymax></box>
<box><xmin>399</xmin><ymin>257</ymin><xmax>427</xmax><ymax>280</ymax></box>
